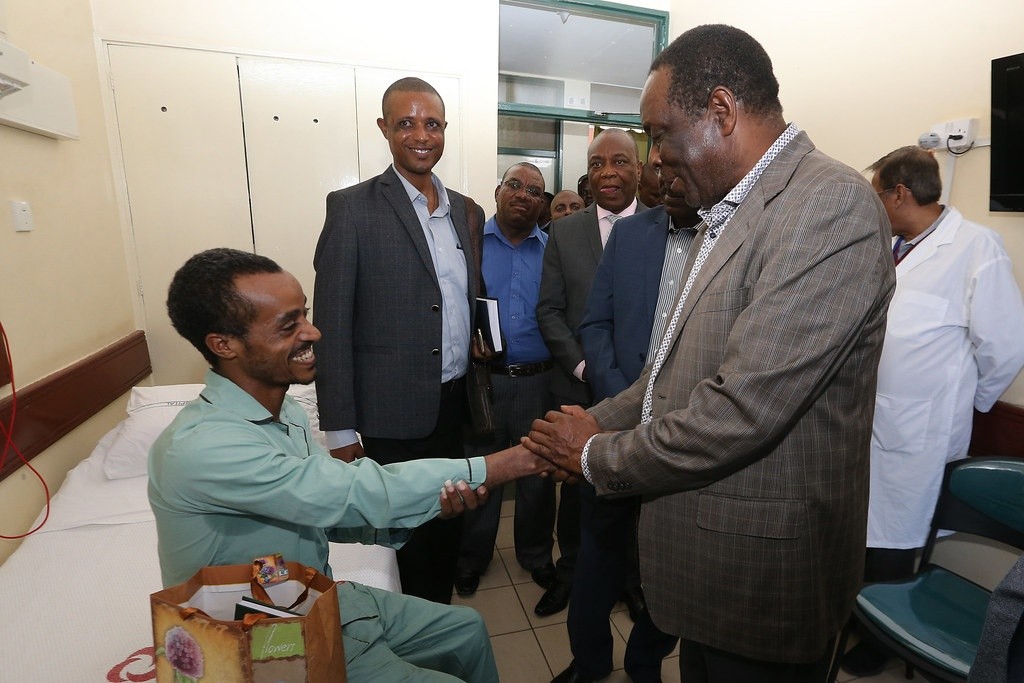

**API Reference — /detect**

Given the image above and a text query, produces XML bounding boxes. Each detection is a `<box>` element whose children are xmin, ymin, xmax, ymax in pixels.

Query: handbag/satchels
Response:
<box><xmin>149</xmin><ymin>553</ymin><xmax>347</xmax><ymax>683</ymax></box>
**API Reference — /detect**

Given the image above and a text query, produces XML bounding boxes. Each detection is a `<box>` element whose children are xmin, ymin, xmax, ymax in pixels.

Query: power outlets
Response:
<box><xmin>931</xmin><ymin>120</ymin><xmax>971</xmax><ymax>151</ymax></box>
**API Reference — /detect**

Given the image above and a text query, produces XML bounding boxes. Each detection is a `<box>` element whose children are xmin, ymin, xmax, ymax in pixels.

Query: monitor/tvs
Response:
<box><xmin>990</xmin><ymin>53</ymin><xmax>1024</xmax><ymax>212</ymax></box>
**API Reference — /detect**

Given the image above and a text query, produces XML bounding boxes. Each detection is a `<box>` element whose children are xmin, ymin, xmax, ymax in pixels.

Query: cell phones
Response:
<box><xmin>478</xmin><ymin>329</ymin><xmax>484</xmax><ymax>354</ymax></box>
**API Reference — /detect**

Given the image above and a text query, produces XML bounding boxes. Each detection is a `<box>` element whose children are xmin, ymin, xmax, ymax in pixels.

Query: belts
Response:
<box><xmin>441</xmin><ymin>375</ymin><xmax>466</xmax><ymax>393</ymax></box>
<box><xmin>490</xmin><ymin>360</ymin><xmax>549</xmax><ymax>378</ymax></box>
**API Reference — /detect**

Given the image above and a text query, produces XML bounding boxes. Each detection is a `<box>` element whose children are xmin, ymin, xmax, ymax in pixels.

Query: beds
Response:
<box><xmin>0</xmin><ymin>389</ymin><xmax>403</xmax><ymax>683</ymax></box>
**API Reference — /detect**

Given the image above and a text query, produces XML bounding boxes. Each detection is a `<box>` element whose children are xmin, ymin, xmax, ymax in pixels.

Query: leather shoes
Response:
<box><xmin>532</xmin><ymin>564</ymin><xmax>556</xmax><ymax>588</ymax></box>
<box><xmin>622</xmin><ymin>582</ymin><xmax>647</xmax><ymax>623</ymax></box>
<box><xmin>535</xmin><ymin>582</ymin><xmax>576</xmax><ymax>616</ymax></box>
<box><xmin>454</xmin><ymin>568</ymin><xmax>479</xmax><ymax>595</ymax></box>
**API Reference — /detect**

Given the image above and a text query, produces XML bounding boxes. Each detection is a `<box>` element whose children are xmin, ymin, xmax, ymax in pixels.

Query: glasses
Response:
<box><xmin>501</xmin><ymin>181</ymin><xmax>545</xmax><ymax>205</ymax></box>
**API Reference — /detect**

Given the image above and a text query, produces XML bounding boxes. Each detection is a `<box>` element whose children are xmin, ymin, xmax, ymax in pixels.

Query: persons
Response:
<box><xmin>471</xmin><ymin>15</ymin><xmax>893</xmax><ymax>683</ymax></box>
<box><xmin>147</xmin><ymin>248</ymin><xmax>551</xmax><ymax>683</ymax></box>
<box><xmin>313</xmin><ymin>75</ymin><xmax>504</xmax><ymax>607</ymax></box>
<box><xmin>841</xmin><ymin>145</ymin><xmax>1024</xmax><ymax>677</ymax></box>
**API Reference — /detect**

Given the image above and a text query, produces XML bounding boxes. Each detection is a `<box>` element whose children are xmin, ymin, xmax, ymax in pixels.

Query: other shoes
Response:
<box><xmin>551</xmin><ymin>658</ymin><xmax>614</xmax><ymax>683</ymax></box>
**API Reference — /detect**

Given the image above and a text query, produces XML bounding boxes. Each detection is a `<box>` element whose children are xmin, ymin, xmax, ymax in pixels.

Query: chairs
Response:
<box><xmin>826</xmin><ymin>454</ymin><xmax>1024</xmax><ymax>683</ymax></box>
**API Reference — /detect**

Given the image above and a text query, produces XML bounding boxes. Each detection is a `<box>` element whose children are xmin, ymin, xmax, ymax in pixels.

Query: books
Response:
<box><xmin>477</xmin><ymin>296</ymin><xmax>503</xmax><ymax>353</ymax></box>
<box><xmin>234</xmin><ymin>597</ymin><xmax>307</xmax><ymax>621</ymax></box>
